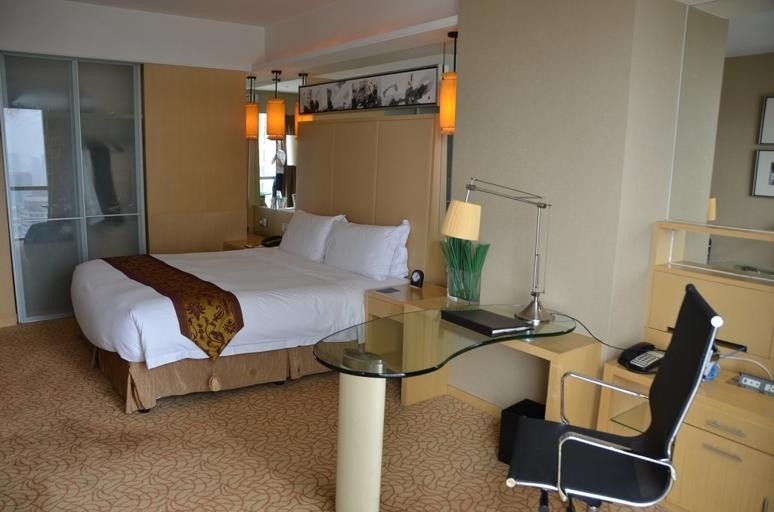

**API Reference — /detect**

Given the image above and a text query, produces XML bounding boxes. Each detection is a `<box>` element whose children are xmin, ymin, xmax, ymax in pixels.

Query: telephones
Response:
<box><xmin>618</xmin><ymin>341</ymin><xmax>666</xmax><ymax>374</ymax></box>
<box><xmin>261</xmin><ymin>235</ymin><xmax>282</xmax><ymax>247</ymax></box>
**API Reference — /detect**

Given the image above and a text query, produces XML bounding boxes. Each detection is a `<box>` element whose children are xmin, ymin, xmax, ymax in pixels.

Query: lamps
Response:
<box><xmin>438</xmin><ymin>31</ymin><xmax>457</xmax><ymax>135</ymax></box>
<box><xmin>243</xmin><ymin>75</ymin><xmax>259</xmax><ymax>140</ymax></box>
<box><xmin>293</xmin><ymin>72</ymin><xmax>314</xmax><ymax>139</ymax></box>
<box><xmin>439</xmin><ymin>176</ymin><xmax>557</xmax><ymax>325</ymax></box>
<box><xmin>266</xmin><ymin>69</ymin><xmax>287</xmax><ymax>140</ymax></box>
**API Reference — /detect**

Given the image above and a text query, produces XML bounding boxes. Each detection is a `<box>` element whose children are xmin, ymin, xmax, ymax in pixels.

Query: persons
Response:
<box><xmin>272</xmin><ymin>142</ymin><xmax>286</xmax><ymax>201</ymax></box>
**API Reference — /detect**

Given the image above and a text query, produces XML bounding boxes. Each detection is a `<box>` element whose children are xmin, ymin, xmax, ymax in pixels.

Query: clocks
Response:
<box><xmin>409</xmin><ymin>270</ymin><xmax>424</xmax><ymax>289</ymax></box>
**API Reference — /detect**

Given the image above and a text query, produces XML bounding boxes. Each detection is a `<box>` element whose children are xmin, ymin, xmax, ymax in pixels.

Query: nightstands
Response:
<box><xmin>364</xmin><ymin>281</ymin><xmax>449</xmax><ymax>406</ymax></box>
<box><xmin>223</xmin><ymin>240</ymin><xmax>264</xmax><ymax>250</ymax></box>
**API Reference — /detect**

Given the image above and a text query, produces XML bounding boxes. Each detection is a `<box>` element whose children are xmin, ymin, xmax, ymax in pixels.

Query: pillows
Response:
<box><xmin>389</xmin><ymin>218</ymin><xmax>411</xmax><ymax>281</ymax></box>
<box><xmin>323</xmin><ymin>220</ymin><xmax>407</xmax><ymax>280</ymax></box>
<box><xmin>277</xmin><ymin>209</ymin><xmax>347</xmax><ymax>265</ymax></box>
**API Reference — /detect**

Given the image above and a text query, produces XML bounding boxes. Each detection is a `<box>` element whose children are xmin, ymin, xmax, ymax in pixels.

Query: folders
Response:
<box><xmin>440</xmin><ymin>310</ymin><xmax>534</xmax><ymax>338</ymax></box>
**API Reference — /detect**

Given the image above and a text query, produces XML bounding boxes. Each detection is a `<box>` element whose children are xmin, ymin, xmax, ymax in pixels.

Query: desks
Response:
<box><xmin>407</xmin><ymin>297</ymin><xmax>601</xmax><ymax>432</ymax></box>
<box><xmin>312</xmin><ymin>302</ymin><xmax>577</xmax><ymax>511</ymax></box>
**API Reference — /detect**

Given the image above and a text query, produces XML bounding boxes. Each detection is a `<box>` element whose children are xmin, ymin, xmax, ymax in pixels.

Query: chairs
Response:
<box><xmin>504</xmin><ymin>282</ymin><xmax>726</xmax><ymax>512</ymax></box>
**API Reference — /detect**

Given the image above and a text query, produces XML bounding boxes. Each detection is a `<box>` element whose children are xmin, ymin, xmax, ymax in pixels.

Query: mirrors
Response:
<box><xmin>247</xmin><ymin>108</ymin><xmax>297</xmax><ymax>211</ymax></box>
<box><xmin>668</xmin><ymin>1</ymin><xmax>774</xmax><ymax>283</ymax></box>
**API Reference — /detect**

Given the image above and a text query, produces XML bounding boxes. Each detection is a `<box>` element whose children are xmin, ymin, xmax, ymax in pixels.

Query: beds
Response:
<box><xmin>68</xmin><ymin>247</ymin><xmax>412</xmax><ymax>415</ymax></box>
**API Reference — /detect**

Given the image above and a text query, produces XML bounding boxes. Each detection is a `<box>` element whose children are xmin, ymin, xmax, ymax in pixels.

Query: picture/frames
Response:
<box><xmin>750</xmin><ymin>148</ymin><xmax>774</xmax><ymax>198</ymax></box>
<box><xmin>756</xmin><ymin>94</ymin><xmax>774</xmax><ymax>146</ymax></box>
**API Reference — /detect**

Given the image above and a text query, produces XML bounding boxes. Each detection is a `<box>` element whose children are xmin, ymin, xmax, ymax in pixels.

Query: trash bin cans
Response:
<box><xmin>499</xmin><ymin>398</ymin><xmax>545</xmax><ymax>465</ymax></box>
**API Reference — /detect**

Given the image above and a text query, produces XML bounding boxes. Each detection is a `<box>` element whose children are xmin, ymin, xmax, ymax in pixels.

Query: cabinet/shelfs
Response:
<box><xmin>594</xmin><ymin>219</ymin><xmax>774</xmax><ymax>512</ymax></box>
